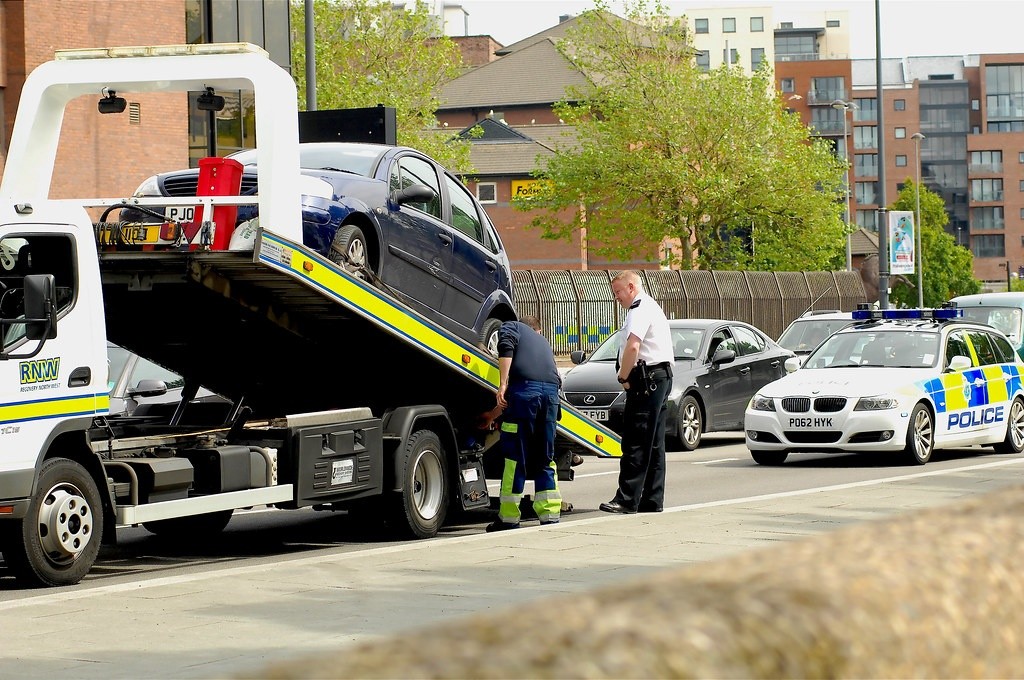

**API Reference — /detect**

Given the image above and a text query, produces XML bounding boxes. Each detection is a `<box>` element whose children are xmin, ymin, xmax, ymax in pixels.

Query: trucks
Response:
<box><xmin>0</xmin><ymin>41</ymin><xmax>631</xmax><ymax>591</ymax></box>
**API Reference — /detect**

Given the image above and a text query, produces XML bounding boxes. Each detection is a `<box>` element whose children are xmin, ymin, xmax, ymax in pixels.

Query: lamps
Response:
<box><xmin>443</xmin><ymin>122</ymin><xmax>450</xmax><ymax>127</ymax></box>
<box><xmin>531</xmin><ymin>119</ymin><xmax>536</xmax><ymax>126</ymax></box>
<box><xmin>559</xmin><ymin>119</ymin><xmax>565</xmax><ymax>124</ymax></box>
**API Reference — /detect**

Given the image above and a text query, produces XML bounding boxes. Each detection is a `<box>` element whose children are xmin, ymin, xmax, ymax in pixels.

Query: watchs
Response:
<box><xmin>618</xmin><ymin>376</ymin><xmax>628</xmax><ymax>384</ymax></box>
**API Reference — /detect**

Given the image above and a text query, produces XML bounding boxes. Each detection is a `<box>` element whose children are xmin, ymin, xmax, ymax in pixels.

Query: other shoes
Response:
<box><xmin>540</xmin><ymin>520</ymin><xmax>558</xmax><ymax>525</ymax></box>
<box><xmin>485</xmin><ymin>515</ymin><xmax>520</xmax><ymax>532</ymax></box>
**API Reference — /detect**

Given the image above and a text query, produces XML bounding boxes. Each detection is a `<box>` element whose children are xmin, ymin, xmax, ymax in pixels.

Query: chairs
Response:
<box><xmin>675</xmin><ymin>340</ymin><xmax>698</xmax><ymax>356</ymax></box>
<box><xmin>701</xmin><ymin>336</ymin><xmax>724</xmax><ymax>363</ymax></box>
<box><xmin>859</xmin><ymin>339</ymin><xmax>887</xmax><ymax>366</ymax></box>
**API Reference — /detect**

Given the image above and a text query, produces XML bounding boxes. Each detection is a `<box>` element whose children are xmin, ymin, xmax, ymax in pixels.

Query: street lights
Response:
<box><xmin>910</xmin><ymin>132</ymin><xmax>925</xmax><ymax>309</ymax></box>
<box><xmin>830</xmin><ymin>99</ymin><xmax>860</xmax><ymax>272</ymax></box>
<box><xmin>1000</xmin><ymin>261</ymin><xmax>1011</xmax><ymax>292</ymax></box>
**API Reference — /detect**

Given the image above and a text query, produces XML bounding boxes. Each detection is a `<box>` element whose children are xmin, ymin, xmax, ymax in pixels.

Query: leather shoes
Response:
<box><xmin>599</xmin><ymin>499</ymin><xmax>638</xmax><ymax>514</ymax></box>
<box><xmin>637</xmin><ymin>497</ymin><xmax>663</xmax><ymax>512</ymax></box>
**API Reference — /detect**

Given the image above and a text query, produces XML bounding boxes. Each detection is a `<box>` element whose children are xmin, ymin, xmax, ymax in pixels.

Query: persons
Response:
<box><xmin>599</xmin><ymin>270</ymin><xmax>675</xmax><ymax>514</ymax></box>
<box><xmin>485</xmin><ymin>315</ymin><xmax>563</xmax><ymax>532</ymax></box>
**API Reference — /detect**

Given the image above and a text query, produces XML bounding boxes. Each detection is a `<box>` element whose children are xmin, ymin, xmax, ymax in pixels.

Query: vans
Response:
<box><xmin>945</xmin><ymin>291</ymin><xmax>1024</xmax><ymax>364</ymax></box>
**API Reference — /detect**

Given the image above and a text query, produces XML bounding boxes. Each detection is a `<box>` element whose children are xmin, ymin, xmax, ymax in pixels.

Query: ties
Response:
<box><xmin>615</xmin><ymin>350</ymin><xmax>620</xmax><ymax>374</ymax></box>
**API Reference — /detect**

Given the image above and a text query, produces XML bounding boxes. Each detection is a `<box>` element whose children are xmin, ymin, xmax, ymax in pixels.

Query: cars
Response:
<box><xmin>117</xmin><ymin>142</ymin><xmax>521</xmax><ymax>360</ymax></box>
<box><xmin>742</xmin><ymin>308</ymin><xmax>1021</xmax><ymax>468</ymax></box>
<box><xmin>558</xmin><ymin>319</ymin><xmax>802</xmax><ymax>453</ymax></box>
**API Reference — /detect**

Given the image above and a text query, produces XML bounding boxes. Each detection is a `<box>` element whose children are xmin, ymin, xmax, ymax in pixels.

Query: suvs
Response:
<box><xmin>775</xmin><ymin>308</ymin><xmax>877</xmax><ymax>370</ymax></box>
